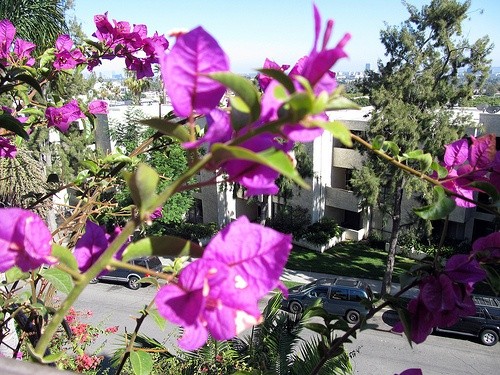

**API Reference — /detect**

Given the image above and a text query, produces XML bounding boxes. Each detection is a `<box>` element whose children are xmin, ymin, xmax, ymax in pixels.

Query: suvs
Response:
<box><xmin>90</xmin><ymin>255</ymin><xmax>163</xmax><ymax>289</ymax></box>
<box><xmin>390</xmin><ymin>293</ymin><xmax>500</xmax><ymax>346</ymax></box>
<box><xmin>279</xmin><ymin>278</ymin><xmax>374</xmax><ymax>324</ymax></box>
